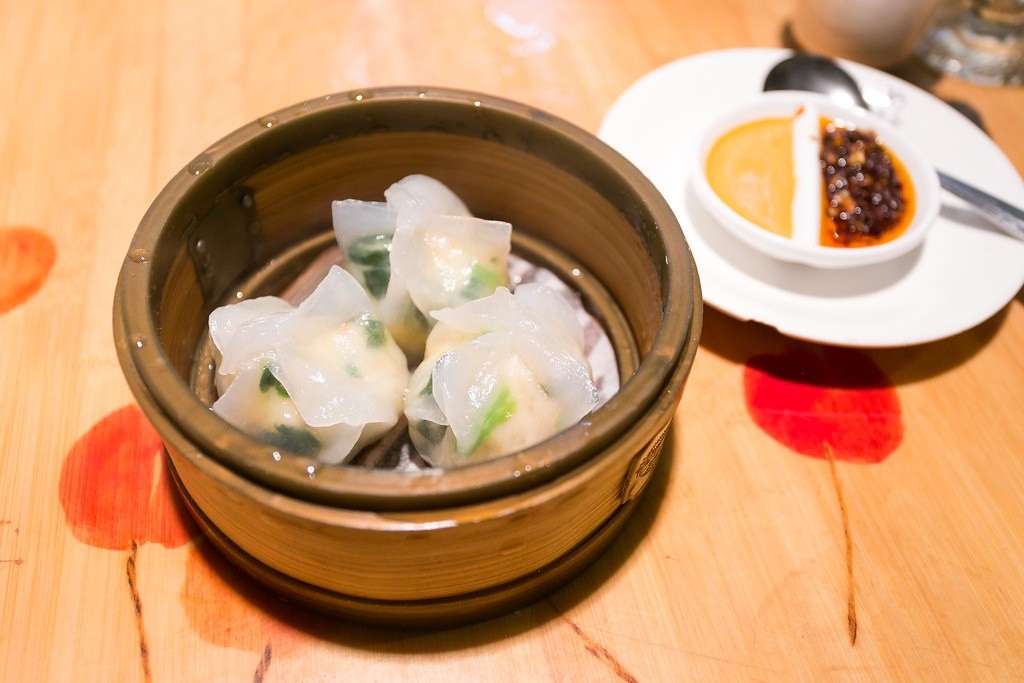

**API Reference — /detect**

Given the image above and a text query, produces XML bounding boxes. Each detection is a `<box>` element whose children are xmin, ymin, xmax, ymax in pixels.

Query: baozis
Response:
<box><xmin>201</xmin><ymin>172</ymin><xmax>599</xmax><ymax>473</ymax></box>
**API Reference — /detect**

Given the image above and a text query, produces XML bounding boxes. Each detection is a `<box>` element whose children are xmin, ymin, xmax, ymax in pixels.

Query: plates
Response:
<box><xmin>594</xmin><ymin>47</ymin><xmax>1024</xmax><ymax>349</ymax></box>
<box><xmin>692</xmin><ymin>90</ymin><xmax>941</xmax><ymax>270</ymax></box>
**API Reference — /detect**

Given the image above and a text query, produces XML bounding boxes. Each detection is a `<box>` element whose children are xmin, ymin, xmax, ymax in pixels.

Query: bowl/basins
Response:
<box><xmin>112</xmin><ymin>87</ymin><xmax>705</xmax><ymax>632</ymax></box>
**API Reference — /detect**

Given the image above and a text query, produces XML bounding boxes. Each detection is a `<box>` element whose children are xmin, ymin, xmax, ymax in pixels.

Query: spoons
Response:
<box><xmin>763</xmin><ymin>55</ymin><xmax>1024</xmax><ymax>242</ymax></box>
